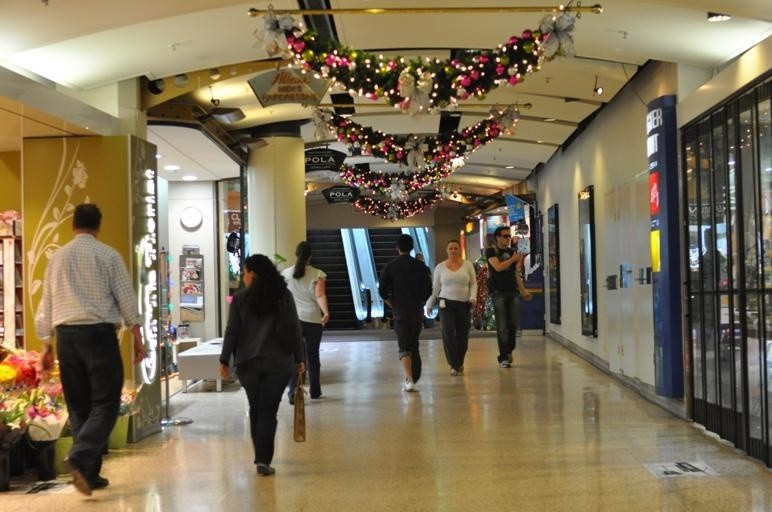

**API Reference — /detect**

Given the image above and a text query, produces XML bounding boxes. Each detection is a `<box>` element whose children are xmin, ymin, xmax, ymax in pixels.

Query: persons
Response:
<box><xmin>221</xmin><ymin>253</ymin><xmax>305</xmax><ymax>474</ymax></box>
<box><xmin>485</xmin><ymin>226</ymin><xmax>532</xmax><ymax>369</ymax></box>
<box><xmin>282</xmin><ymin>241</ymin><xmax>329</xmax><ymax>404</ymax></box>
<box><xmin>414</xmin><ymin>253</ymin><xmax>425</xmax><ymax>261</ymax></box>
<box><xmin>426</xmin><ymin>240</ymin><xmax>477</xmax><ymax>376</ymax></box>
<box><xmin>377</xmin><ymin>234</ymin><xmax>432</xmax><ymax>392</ymax></box>
<box><xmin>33</xmin><ymin>203</ymin><xmax>146</xmax><ymax>497</ymax></box>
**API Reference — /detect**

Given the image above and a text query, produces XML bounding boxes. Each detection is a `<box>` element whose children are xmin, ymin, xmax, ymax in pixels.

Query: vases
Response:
<box><xmin>32</xmin><ymin>441</ymin><xmax>59</xmax><ymax>481</ymax></box>
<box><xmin>54</xmin><ymin>437</ymin><xmax>74</xmax><ymax>474</ymax></box>
<box><xmin>0</xmin><ymin>452</ymin><xmax>10</xmax><ymax>493</ymax></box>
<box><xmin>9</xmin><ymin>440</ymin><xmax>26</xmax><ymax>477</ymax></box>
<box><xmin>107</xmin><ymin>416</ymin><xmax>129</xmax><ymax>449</ymax></box>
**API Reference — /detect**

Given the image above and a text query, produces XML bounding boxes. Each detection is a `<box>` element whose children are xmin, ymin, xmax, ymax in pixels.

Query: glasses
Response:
<box><xmin>497</xmin><ymin>234</ymin><xmax>511</xmax><ymax>238</ymax></box>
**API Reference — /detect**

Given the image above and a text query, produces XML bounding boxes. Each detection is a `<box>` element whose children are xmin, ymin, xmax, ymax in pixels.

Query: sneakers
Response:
<box><xmin>499</xmin><ymin>360</ymin><xmax>510</xmax><ymax>368</ymax></box>
<box><xmin>507</xmin><ymin>353</ymin><xmax>513</xmax><ymax>363</ymax></box>
<box><xmin>457</xmin><ymin>365</ymin><xmax>464</xmax><ymax>375</ymax></box>
<box><xmin>405</xmin><ymin>379</ymin><xmax>413</xmax><ymax>391</ymax></box>
<box><xmin>451</xmin><ymin>368</ymin><xmax>457</xmax><ymax>375</ymax></box>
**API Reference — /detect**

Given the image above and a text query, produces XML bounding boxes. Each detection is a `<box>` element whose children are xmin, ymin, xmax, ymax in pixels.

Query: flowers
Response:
<box><xmin>27</xmin><ymin>393</ymin><xmax>70</xmax><ymax>443</ymax></box>
<box><xmin>118</xmin><ymin>384</ymin><xmax>142</xmax><ymax>416</ymax></box>
<box><xmin>0</xmin><ymin>391</ymin><xmax>37</xmax><ymax>449</ymax></box>
<box><xmin>1</xmin><ymin>343</ymin><xmax>42</xmax><ymax>388</ymax></box>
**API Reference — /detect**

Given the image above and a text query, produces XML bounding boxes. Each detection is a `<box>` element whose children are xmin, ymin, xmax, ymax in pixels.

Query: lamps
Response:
<box><xmin>593</xmin><ymin>74</ymin><xmax>604</xmax><ymax>95</ymax></box>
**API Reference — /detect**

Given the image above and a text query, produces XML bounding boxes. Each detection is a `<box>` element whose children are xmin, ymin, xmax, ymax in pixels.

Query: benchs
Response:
<box><xmin>176</xmin><ymin>336</ymin><xmax>234</xmax><ymax>394</ymax></box>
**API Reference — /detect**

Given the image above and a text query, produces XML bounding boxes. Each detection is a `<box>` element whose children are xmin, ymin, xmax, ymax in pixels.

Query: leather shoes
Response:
<box><xmin>63</xmin><ymin>456</ymin><xmax>92</xmax><ymax>496</ymax></box>
<box><xmin>310</xmin><ymin>390</ymin><xmax>321</xmax><ymax>399</ymax></box>
<box><xmin>290</xmin><ymin>394</ymin><xmax>294</xmax><ymax>405</ymax></box>
<box><xmin>254</xmin><ymin>459</ymin><xmax>258</xmax><ymax>464</ymax></box>
<box><xmin>90</xmin><ymin>475</ymin><xmax>108</xmax><ymax>489</ymax></box>
<box><xmin>257</xmin><ymin>463</ymin><xmax>274</xmax><ymax>474</ymax></box>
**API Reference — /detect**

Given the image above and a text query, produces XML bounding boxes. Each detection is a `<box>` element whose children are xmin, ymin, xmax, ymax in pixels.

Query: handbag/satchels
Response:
<box><xmin>294</xmin><ymin>372</ymin><xmax>305</xmax><ymax>441</ymax></box>
